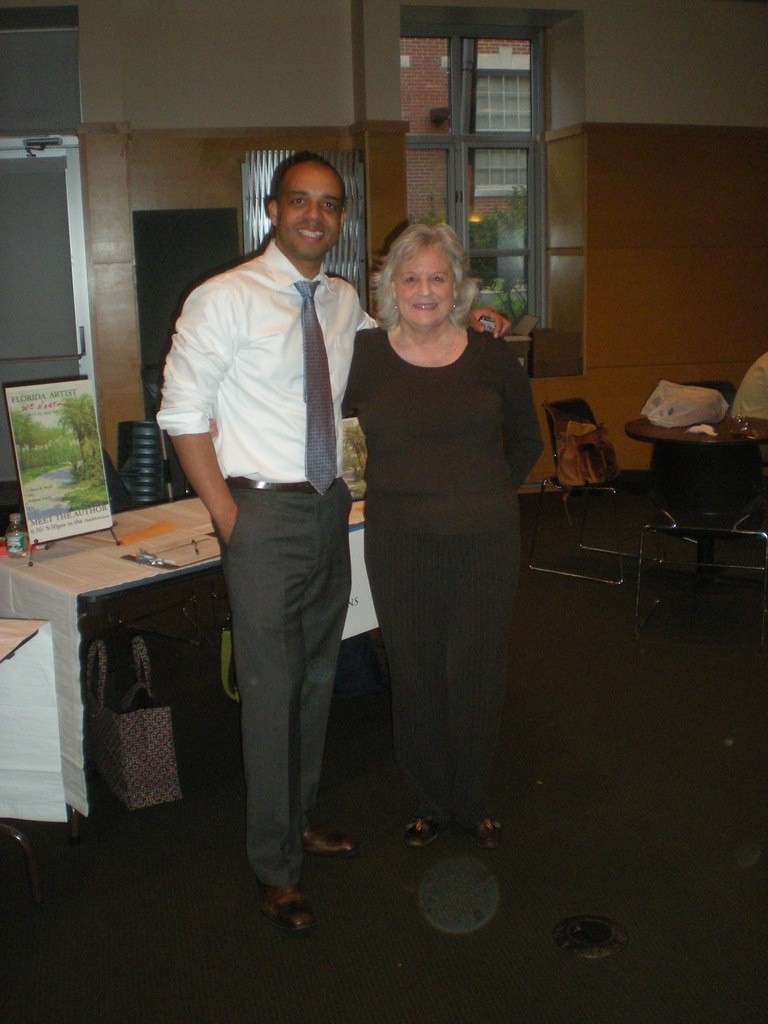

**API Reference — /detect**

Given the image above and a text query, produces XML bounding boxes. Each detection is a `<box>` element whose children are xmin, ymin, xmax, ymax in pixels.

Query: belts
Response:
<box><xmin>225</xmin><ymin>477</ymin><xmax>336</xmax><ymax>494</ymax></box>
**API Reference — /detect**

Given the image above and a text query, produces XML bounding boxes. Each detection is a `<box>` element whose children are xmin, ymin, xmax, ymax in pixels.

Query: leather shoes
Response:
<box><xmin>258</xmin><ymin>879</ymin><xmax>317</xmax><ymax>932</ymax></box>
<box><xmin>300</xmin><ymin>825</ymin><xmax>359</xmax><ymax>857</ymax></box>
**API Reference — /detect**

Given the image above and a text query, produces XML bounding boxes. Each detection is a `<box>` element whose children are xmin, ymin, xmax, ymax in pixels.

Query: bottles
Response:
<box><xmin>5</xmin><ymin>513</ymin><xmax>29</xmax><ymax>558</ymax></box>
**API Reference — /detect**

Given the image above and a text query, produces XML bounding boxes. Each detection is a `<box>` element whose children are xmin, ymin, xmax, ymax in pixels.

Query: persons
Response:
<box><xmin>729</xmin><ymin>349</ymin><xmax>768</xmax><ymax>468</ymax></box>
<box><xmin>154</xmin><ymin>150</ymin><xmax>515</xmax><ymax>933</ymax></box>
<box><xmin>344</xmin><ymin>222</ymin><xmax>546</xmax><ymax>850</ymax></box>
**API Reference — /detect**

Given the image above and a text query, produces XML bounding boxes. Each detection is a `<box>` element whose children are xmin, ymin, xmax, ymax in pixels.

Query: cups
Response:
<box><xmin>728</xmin><ymin>408</ymin><xmax>754</xmax><ymax>437</ymax></box>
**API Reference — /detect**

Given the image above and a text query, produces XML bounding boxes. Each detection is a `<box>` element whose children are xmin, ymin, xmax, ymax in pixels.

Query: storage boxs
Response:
<box><xmin>503</xmin><ymin>314</ymin><xmax>542</xmax><ymax>376</ymax></box>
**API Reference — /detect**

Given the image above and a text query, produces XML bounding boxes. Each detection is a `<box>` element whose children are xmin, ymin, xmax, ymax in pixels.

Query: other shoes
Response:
<box><xmin>473</xmin><ymin>818</ymin><xmax>500</xmax><ymax>850</ymax></box>
<box><xmin>405</xmin><ymin>815</ymin><xmax>444</xmax><ymax>847</ymax></box>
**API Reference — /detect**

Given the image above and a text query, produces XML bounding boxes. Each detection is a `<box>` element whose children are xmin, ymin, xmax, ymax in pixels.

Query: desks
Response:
<box><xmin>624</xmin><ymin>415</ymin><xmax>768</xmax><ymax>597</ymax></box>
<box><xmin>0</xmin><ymin>494</ymin><xmax>378</xmax><ymax>907</ymax></box>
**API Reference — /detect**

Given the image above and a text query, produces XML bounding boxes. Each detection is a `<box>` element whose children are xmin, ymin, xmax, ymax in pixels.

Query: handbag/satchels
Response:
<box><xmin>557</xmin><ymin>420</ymin><xmax>620</xmax><ymax>486</ymax></box>
<box><xmin>642</xmin><ymin>380</ymin><xmax>730</xmax><ymax>428</ymax></box>
<box><xmin>85</xmin><ymin>635</ymin><xmax>182</xmax><ymax>809</ymax></box>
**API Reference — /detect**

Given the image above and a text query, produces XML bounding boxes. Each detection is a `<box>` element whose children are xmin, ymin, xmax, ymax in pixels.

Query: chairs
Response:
<box><xmin>633</xmin><ymin>439</ymin><xmax>768</xmax><ymax>652</ymax></box>
<box><xmin>528</xmin><ymin>398</ymin><xmax>650</xmax><ymax>586</ymax></box>
<box><xmin>680</xmin><ymin>380</ymin><xmax>737</xmax><ymax>419</ymax></box>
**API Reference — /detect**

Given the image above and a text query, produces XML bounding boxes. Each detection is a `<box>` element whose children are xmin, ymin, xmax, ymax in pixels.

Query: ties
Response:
<box><xmin>294</xmin><ymin>281</ymin><xmax>337</xmax><ymax>495</ymax></box>
